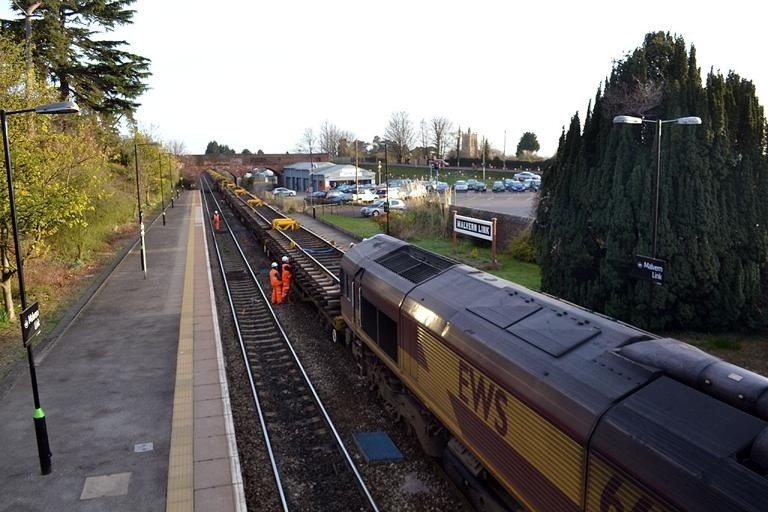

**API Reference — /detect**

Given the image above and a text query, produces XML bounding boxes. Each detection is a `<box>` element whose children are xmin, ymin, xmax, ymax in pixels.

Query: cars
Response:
<box><xmin>386</xmin><ymin>176</ymin><xmax>449</xmax><ymax>194</ymax></box>
<box><xmin>360</xmin><ymin>200</ymin><xmax>405</xmax><ymax>217</ymax></box>
<box><xmin>514</xmin><ymin>171</ymin><xmax>541</xmax><ymax>179</ymax></box>
<box><xmin>428</xmin><ymin>159</ymin><xmax>450</xmax><ymax>168</ymax></box>
<box><xmin>304</xmin><ymin>184</ymin><xmax>379</xmax><ymax>203</ymax></box>
<box><xmin>270</xmin><ymin>187</ymin><xmax>297</xmax><ymax>197</ymax></box>
<box><xmin>455</xmin><ymin>177</ymin><xmax>541</xmax><ymax>192</ymax></box>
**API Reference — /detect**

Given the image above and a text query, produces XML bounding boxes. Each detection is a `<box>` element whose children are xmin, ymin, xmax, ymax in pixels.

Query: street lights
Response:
<box><xmin>612</xmin><ymin>114</ymin><xmax>703</xmax><ymax>329</ymax></box>
<box><xmin>0</xmin><ymin>100</ymin><xmax>79</xmax><ymax>475</ymax></box>
<box><xmin>134</xmin><ymin>141</ymin><xmax>184</xmax><ymax>279</ymax></box>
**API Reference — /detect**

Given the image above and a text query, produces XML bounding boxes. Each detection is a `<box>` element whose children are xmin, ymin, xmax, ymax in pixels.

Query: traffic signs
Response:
<box><xmin>17</xmin><ymin>302</ymin><xmax>43</xmax><ymax>347</ymax></box>
<box><xmin>634</xmin><ymin>254</ymin><xmax>665</xmax><ymax>284</ymax></box>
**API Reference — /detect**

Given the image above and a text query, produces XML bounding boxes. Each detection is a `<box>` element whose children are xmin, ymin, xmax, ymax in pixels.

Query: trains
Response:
<box><xmin>337</xmin><ymin>234</ymin><xmax>767</xmax><ymax>510</ymax></box>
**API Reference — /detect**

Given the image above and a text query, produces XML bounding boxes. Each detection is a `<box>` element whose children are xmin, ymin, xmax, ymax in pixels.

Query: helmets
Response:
<box><xmin>271</xmin><ymin>262</ymin><xmax>279</xmax><ymax>267</ymax></box>
<box><xmin>282</xmin><ymin>256</ymin><xmax>287</xmax><ymax>261</ymax></box>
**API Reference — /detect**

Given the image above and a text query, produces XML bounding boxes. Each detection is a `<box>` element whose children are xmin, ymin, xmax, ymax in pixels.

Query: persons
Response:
<box><xmin>269</xmin><ymin>261</ymin><xmax>282</xmax><ymax>305</ymax></box>
<box><xmin>212</xmin><ymin>210</ymin><xmax>221</xmax><ymax>231</ymax></box>
<box><xmin>282</xmin><ymin>256</ymin><xmax>294</xmax><ymax>303</ymax></box>
